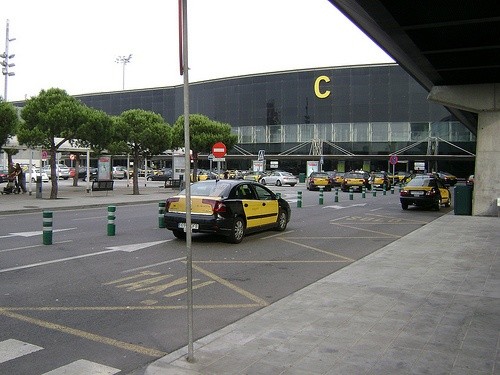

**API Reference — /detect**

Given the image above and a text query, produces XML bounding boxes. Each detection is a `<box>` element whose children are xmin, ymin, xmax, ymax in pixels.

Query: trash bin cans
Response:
<box><xmin>299</xmin><ymin>172</ymin><xmax>305</xmax><ymax>184</ymax></box>
<box><xmin>453</xmin><ymin>182</ymin><xmax>473</xmax><ymax>215</ymax></box>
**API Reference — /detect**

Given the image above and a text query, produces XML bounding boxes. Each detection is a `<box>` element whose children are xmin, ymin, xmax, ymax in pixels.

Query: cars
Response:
<box><xmin>69</xmin><ymin>165</ymin><xmax>251</xmax><ymax>181</ymax></box>
<box><xmin>161</xmin><ymin>179</ymin><xmax>292</xmax><ymax>242</ymax></box>
<box><xmin>399</xmin><ymin>173</ymin><xmax>452</xmax><ymax>209</ymax></box>
<box><xmin>40</xmin><ymin>163</ymin><xmax>71</xmax><ymax>179</ymax></box>
<box><xmin>367</xmin><ymin>172</ymin><xmax>391</xmax><ymax>191</ymax></box>
<box><xmin>260</xmin><ymin>170</ymin><xmax>297</xmax><ymax>187</ymax></box>
<box><xmin>340</xmin><ymin>171</ymin><xmax>370</xmax><ymax>192</ymax></box>
<box><xmin>329</xmin><ymin>171</ymin><xmax>346</xmax><ymax>186</ymax></box>
<box><xmin>306</xmin><ymin>172</ymin><xmax>332</xmax><ymax>191</ymax></box>
<box><xmin>16</xmin><ymin>168</ymin><xmax>50</xmax><ymax>183</ymax></box>
<box><xmin>0</xmin><ymin>170</ymin><xmax>17</xmax><ymax>182</ymax></box>
<box><xmin>432</xmin><ymin>172</ymin><xmax>458</xmax><ymax>187</ymax></box>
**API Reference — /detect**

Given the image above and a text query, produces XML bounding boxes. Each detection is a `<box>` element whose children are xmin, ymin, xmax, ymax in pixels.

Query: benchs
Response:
<box><xmin>161</xmin><ymin>180</ymin><xmax>181</xmax><ymax>195</ymax></box>
<box><xmin>90</xmin><ymin>181</ymin><xmax>114</xmax><ymax>197</ymax></box>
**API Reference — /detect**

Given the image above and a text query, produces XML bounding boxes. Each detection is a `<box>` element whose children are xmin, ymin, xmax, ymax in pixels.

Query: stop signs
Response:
<box><xmin>212</xmin><ymin>142</ymin><xmax>226</xmax><ymax>158</ymax></box>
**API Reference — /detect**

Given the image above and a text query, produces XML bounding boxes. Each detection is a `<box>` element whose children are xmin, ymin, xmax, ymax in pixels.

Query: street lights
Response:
<box><xmin>0</xmin><ymin>18</ymin><xmax>17</xmax><ymax>102</ymax></box>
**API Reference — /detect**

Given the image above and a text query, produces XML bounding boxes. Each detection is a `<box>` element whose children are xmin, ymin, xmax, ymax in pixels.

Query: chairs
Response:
<box><xmin>238</xmin><ymin>189</ymin><xmax>252</xmax><ymax>199</ymax></box>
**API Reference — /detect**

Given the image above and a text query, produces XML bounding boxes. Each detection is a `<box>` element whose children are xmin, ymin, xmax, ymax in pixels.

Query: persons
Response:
<box><xmin>6</xmin><ymin>162</ymin><xmax>27</xmax><ymax>194</ymax></box>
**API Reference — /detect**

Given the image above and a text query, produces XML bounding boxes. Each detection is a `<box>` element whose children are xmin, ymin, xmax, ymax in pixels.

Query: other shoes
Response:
<box><xmin>22</xmin><ymin>191</ymin><xmax>27</xmax><ymax>194</ymax></box>
